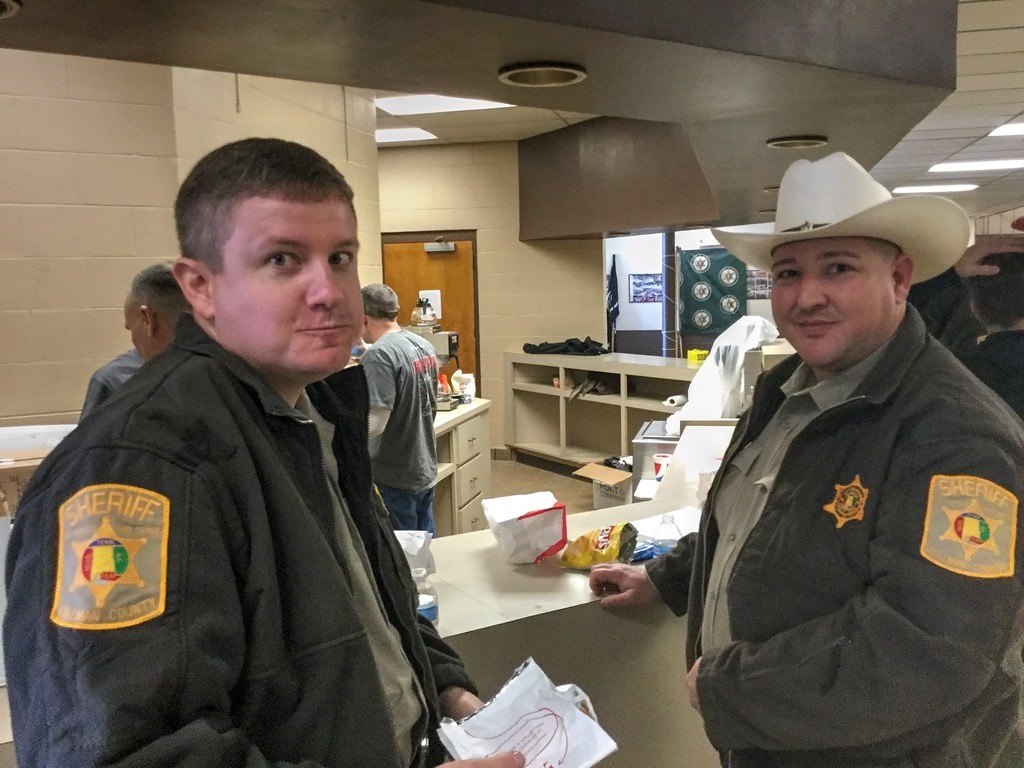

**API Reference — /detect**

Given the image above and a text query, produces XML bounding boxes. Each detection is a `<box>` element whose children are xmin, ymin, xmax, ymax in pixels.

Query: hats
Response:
<box><xmin>711</xmin><ymin>151</ymin><xmax>969</xmax><ymax>287</ymax></box>
<box><xmin>363</xmin><ymin>283</ymin><xmax>400</xmax><ymax>318</ymax></box>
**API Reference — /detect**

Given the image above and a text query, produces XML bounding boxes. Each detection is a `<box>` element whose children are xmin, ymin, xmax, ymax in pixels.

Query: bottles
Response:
<box><xmin>411</xmin><ymin>568</ymin><xmax>438</xmax><ymax>634</ymax></box>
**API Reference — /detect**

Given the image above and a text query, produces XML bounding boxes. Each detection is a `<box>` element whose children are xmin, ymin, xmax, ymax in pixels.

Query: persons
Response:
<box><xmin>2</xmin><ymin>137</ymin><xmax>526</xmax><ymax>768</ymax></box>
<box><xmin>590</xmin><ymin>151</ymin><xmax>1024</xmax><ymax>768</ymax></box>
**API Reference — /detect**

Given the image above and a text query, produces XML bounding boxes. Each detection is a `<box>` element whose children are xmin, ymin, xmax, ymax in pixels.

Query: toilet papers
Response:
<box><xmin>661</xmin><ymin>396</ymin><xmax>687</xmax><ymax>407</ymax></box>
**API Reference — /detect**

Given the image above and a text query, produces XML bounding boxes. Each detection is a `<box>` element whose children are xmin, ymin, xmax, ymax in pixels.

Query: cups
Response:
<box><xmin>653</xmin><ymin>454</ymin><xmax>672</xmax><ymax>482</ymax></box>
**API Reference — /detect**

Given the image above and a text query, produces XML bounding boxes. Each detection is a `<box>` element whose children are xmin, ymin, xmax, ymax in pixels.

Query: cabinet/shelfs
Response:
<box><xmin>504</xmin><ymin>352</ymin><xmax>708</xmax><ymax>467</ymax></box>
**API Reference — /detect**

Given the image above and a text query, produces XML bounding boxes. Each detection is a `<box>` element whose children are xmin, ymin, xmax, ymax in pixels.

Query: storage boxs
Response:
<box><xmin>572</xmin><ymin>461</ymin><xmax>633</xmax><ymax>509</ymax></box>
<box><xmin>431</xmin><ymin>394</ymin><xmax>489</xmax><ymax>532</ymax></box>
